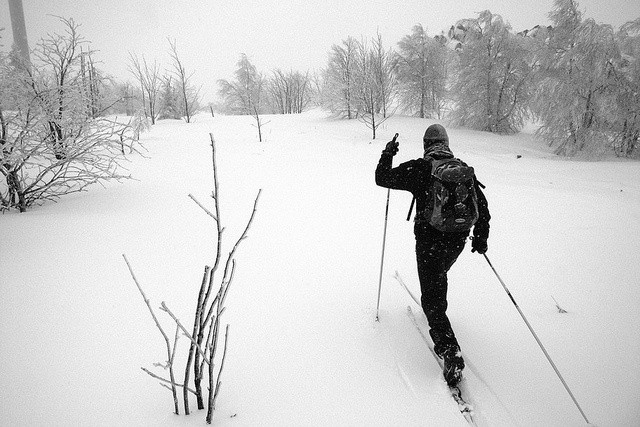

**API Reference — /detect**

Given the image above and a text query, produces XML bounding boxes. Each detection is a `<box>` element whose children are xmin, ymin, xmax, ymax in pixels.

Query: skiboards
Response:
<box><xmin>393</xmin><ymin>270</ymin><xmax>477</xmax><ymax>427</ymax></box>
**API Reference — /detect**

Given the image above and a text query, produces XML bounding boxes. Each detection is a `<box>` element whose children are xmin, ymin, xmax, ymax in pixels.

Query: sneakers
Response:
<box><xmin>433</xmin><ymin>337</ymin><xmax>446</xmax><ymax>357</ymax></box>
<box><xmin>444</xmin><ymin>343</ymin><xmax>466</xmax><ymax>387</ymax></box>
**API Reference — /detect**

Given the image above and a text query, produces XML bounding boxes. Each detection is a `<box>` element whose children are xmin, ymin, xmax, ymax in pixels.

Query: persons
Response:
<box><xmin>375</xmin><ymin>123</ymin><xmax>491</xmax><ymax>388</ymax></box>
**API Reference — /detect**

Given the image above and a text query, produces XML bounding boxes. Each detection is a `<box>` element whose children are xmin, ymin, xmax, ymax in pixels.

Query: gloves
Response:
<box><xmin>472</xmin><ymin>234</ymin><xmax>488</xmax><ymax>255</ymax></box>
<box><xmin>384</xmin><ymin>139</ymin><xmax>400</xmax><ymax>155</ymax></box>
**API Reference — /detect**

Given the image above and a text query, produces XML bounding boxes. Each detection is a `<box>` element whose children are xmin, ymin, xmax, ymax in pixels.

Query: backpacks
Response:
<box><xmin>421</xmin><ymin>155</ymin><xmax>480</xmax><ymax>236</ymax></box>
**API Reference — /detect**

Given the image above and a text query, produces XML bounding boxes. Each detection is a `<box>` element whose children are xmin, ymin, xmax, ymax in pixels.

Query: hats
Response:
<box><xmin>422</xmin><ymin>124</ymin><xmax>448</xmax><ymax>147</ymax></box>
